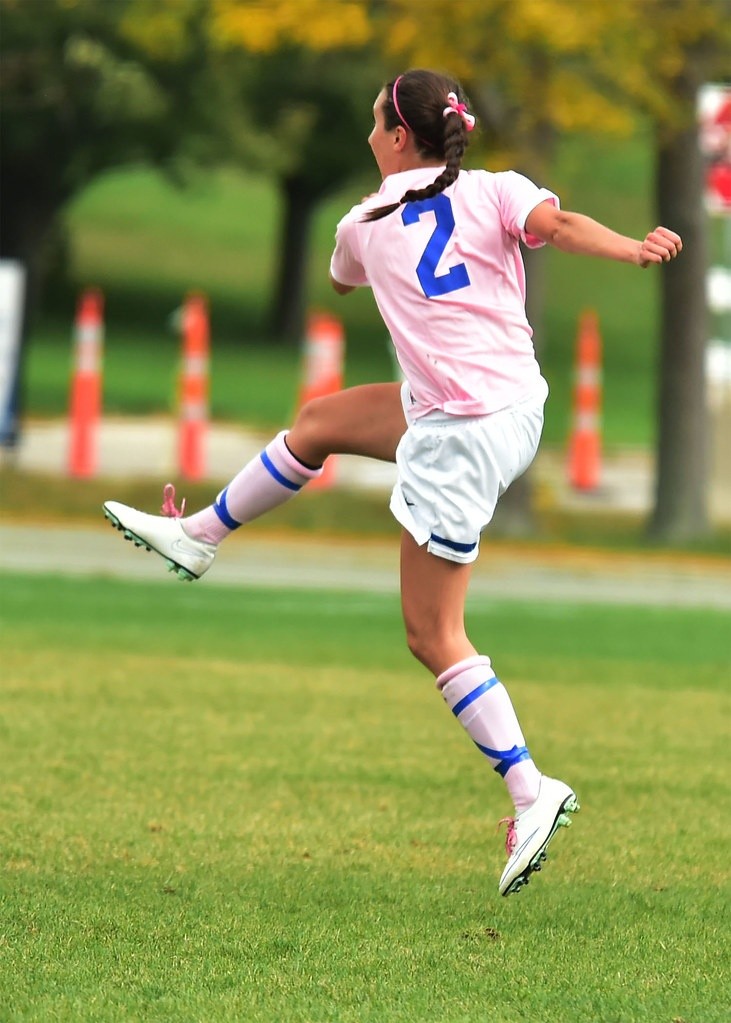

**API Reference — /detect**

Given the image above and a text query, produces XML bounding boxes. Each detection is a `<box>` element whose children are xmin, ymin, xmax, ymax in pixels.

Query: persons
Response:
<box><xmin>101</xmin><ymin>70</ymin><xmax>682</xmax><ymax>897</ymax></box>
<box><xmin>0</xmin><ymin>221</ymin><xmax>78</xmax><ymax>449</ymax></box>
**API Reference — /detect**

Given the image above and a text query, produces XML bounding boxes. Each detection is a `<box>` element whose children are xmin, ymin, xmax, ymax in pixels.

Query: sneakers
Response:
<box><xmin>102</xmin><ymin>484</ymin><xmax>217</xmax><ymax>581</ymax></box>
<box><xmin>498</xmin><ymin>774</ymin><xmax>581</xmax><ymax>898</ymax></box>
